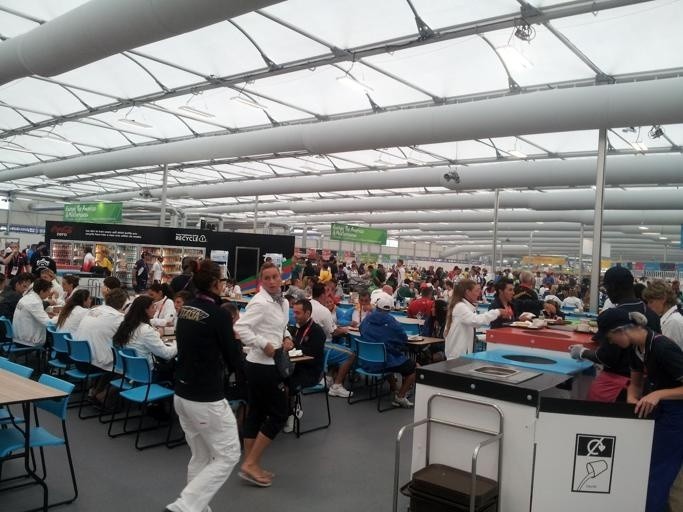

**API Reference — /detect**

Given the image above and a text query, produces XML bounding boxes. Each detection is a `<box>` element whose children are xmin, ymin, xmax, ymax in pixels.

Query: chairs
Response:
<box><xmin>0</xmin><ymin>356</ymin><xmax>36</xmax><ymax>482</ymax></box>
<box><xmin>99</xmin><ymin>346</ymin><xmax>136</xmax><ymax>424</ymax></box>
<box><xmin>293</xmin><ymin>348</ymin><xmax>332</xmax><ymax>439</ymax></box>
<box><xmin>0</xmin><ymin>368</ymin><xmax>78</xmax><ymax>512</ymax></box>
<box><xmin>348</xmin><ymin>338</ymin><xmax>402</xmax><ymax>413</ymax></box>
<box><xmin>399</xmin><ymin>322</ymin><xmax>421</xmax><ymax>336</ymax></box>
<box><xmin>47</xmin><ymin>326</ymin><xmax>73</xmax><ymax>380</ymax></box>
<box><xmin>64</xmin><ymin>335</ymin><xmax>112</xmax><ymax>420</ymax></box>
<box><xmin>0</xmin><ymin>315</ymin><xmax>41</xmax><ymax>382</ymax></box>
<box><xmin>107</xmin><ymin>350</ymin><xmax>176</xmax><ymax>451</ymax></box>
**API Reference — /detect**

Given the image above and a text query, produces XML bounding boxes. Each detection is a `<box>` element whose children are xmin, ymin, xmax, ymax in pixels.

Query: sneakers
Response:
<box><xmin>49</xmin><ymin>367</ymin><xmax>66</xmax><ymax>377</ymax></box>
<box><xmin>283</xmin><ymin>407</ymin><xmax>303</xmax><ymax>433</ymax></box>
<box><xmin>319</xmin><ymin>375</ymin><xmax>333</xmax><ymax>388</ymax></box>
<box><xmin>392</xmin><ymin>393</ymin><xmax>414</xmax><ymax>408</ymax></box>
<box><xmin>328</xmin><ymin>383</ymin><xmax>354</xmax><ymax>398</ymax></box>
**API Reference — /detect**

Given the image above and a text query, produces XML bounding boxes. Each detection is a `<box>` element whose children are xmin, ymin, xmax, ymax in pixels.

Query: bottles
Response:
<box><xmin>395</xmin><ymin>300</ymin><xmax>401</xmax><ymax>309</ymax></box>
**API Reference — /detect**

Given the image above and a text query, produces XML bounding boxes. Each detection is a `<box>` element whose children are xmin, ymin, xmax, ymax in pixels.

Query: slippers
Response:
<box><xmin>93</xmin><ymin>405</ymin><xmax>119</xmax><ymax>413</ymax></box>
<box><xmin>87</xmin><ymin>398</ymin><xmax>103</xmax><ymax>407</ymax></box>
<box><xmin>238</xmin><ymin>472</ymin><xmax>271</xmax><ymax>487</ymax></box>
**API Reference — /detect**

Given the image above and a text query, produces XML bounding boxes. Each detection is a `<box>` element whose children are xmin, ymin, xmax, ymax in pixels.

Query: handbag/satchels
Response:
<box><xmin>273</xmin><ymin>347</ymin><xmax>295</xmax><ymax>378</ymax></box>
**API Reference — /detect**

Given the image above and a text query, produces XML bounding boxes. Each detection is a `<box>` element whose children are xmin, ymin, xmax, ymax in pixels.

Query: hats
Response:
<box><xmin>598</xmin><ymin>267</ymin><xmax>633</xmax><ymax>286</ymax></box>
<box><xmin>378</xmin><ymin>295</ymin><xmax>394</xmax><ymax>311</ymax></box>
<box><xmin>590</xmin><ymin>308</ymin><xmax>633</xmax><ymax>341</ymax></box>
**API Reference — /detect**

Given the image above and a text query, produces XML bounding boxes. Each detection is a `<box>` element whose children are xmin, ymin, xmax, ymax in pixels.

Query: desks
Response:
<box><xmin>289</xmin><ymin>355</ymin><xmax>315</xmax><ymax>363</ymax></box>
<box><xmin>0</xmin><ymin>368</ymin><xmax>69</xmax><ymax>406</ymax></box>
<box><xmin>344</xmin><ymin>327</ymin><xmax>445</xmax><ymax>407</ymax></box>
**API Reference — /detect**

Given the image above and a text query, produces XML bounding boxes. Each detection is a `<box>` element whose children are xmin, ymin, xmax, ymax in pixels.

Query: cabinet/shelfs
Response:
<box><xmin>46</xmin><ymin>220</ymin><xmax>207</xmax><ymax>290</ymax></box>
<box><xmin>409</xmin><ymin>357</ymin><xmax>655</xmax><ymax>512</ymax></box>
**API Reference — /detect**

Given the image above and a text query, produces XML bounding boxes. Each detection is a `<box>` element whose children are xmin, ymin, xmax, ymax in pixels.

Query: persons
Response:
<box><xmin>592</xmin><ymin>307</ymin><xmax>683</xmax><ymax>512</ymax></box>
<box><xmin>232</xmin><ymin>262</ymin><xmax>294</xmax><ymax>487</ymax></box>
<box><xmin>165</xmin><ymin>259</ymin><xmax>248</xmax><ymax>511</ymax></box>
<box><xmin>0</xmin><ymin>244</ymin><xmax>683</xmax><ymax>435</ymax></box>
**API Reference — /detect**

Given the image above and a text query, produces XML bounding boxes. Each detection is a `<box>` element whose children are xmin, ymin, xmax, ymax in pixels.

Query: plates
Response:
<box><xmin>288</xmin><ymin>349</ymin><xmax>303</xmax><ymax>357</ymax></box>
<box><xmin>547</xmin><ymin>319</ymin><xmax>572</xmax><ymax>326</ymax></box>
<box><xmin>408</xmin><ymin>337</ymin><xmax>425</xmax><ymax>343</ymax></box>
<box><xmin>503</xmin><ymin>321</ymin><xmax>530</xmax><ymax>329</ymax></box>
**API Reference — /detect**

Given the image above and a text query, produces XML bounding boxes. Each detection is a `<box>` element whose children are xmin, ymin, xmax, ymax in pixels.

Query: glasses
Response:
<box><xmin>219</xmin><ymin>277</ymin><xmax>231</xmax><ymax>284</ymax></box>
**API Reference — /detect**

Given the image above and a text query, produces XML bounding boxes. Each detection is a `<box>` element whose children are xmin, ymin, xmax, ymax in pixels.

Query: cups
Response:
<box><xmin>165</xmin><ymin>326</ymin><xmax>175</xmax><ymax>335</ymax></box>
<box><xmin>586</xmin><ymin>460</ymin><xmax>608</xmax><ymax>478</ymax></box>
<box><xmin>581</xmin><ymin>319</ymin><xmax>589</xmax><ymax>325</ymax></box>
<box><xmin>343</xmin><ymin>295</ymin><xmax>349</xmax><ymax>304</ymax></box>
<box><xmin>578</xmin><ymin>326</ymin><xmax>589</xmax><ymax>333</ymax></box>
<box><xmin>236</xmin><ymin>291</ymin><xmax>243</xmax><ymax>301</ymax></box>
<box><xmin>574</xmin><ymin>309</ymin><xmax>579</xmax><ymax>313</ymax></box>
<box><xmin>533</xmin><ymin>319</ymin><xmax>544</xmax><ymax>327</ymax></box>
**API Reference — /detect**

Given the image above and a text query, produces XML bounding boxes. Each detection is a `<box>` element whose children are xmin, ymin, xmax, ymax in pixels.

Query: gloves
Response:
<box><xmin>569</xmin><ymin>344</ymin><xmax>590</xmax><ymax>361</ymax></box>
